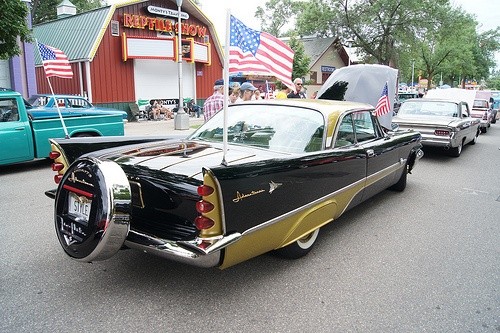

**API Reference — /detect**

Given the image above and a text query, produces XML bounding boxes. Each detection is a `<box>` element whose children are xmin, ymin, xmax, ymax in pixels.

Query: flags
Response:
<box><xmin>229</xmin><ymin>14</ymin><xmax>296</xmax><ymax>92</ymax></box>
<box><xmin>376</xmin><ymin>82</ymin><xmax>391</xmax><ymax>117</ymax></box>
<box><xmin>36</xmin><ymin>42</ymin><xmax>74</xmax><ymax>78</ymax></box>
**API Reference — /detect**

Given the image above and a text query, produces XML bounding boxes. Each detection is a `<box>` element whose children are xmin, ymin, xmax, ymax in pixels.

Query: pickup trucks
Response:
<box><xmin>0</xmin><ymin>85</ymin><xmax>130</xmax><ymax>169</ymax></box>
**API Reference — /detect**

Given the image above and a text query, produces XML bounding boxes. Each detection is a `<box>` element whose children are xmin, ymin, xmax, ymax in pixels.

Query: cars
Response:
<box><xmin>43</xmin><ymin>62</ymin><xmax>424</xmax><ymax>272</ymax></box>
<box><xmin>391</xmin><ymin>85</ymin><xmax>500</xmax><ymax>157</ymax></box>
<box><xmin>24</xmin><ymin>93</ymin><xmax>129</xmax><ymax>125</ymax></box>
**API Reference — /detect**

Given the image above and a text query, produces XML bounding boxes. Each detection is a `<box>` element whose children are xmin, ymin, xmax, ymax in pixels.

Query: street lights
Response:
<box><xmin>410</xmin><ymin>59</ymin><xmax>415</xmax><ymax>89</ymax></box>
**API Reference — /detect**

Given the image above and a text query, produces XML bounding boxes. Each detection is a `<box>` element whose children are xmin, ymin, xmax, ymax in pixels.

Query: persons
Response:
<box><xmin>148</xmin><ymin>78</ymin><xmax>317</xmax><ymax>125</ymax></box>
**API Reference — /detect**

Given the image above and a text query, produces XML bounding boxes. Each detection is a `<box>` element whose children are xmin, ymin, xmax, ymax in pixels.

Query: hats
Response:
<box><xmin>215</xmin><ymin>80</ymin><xmax>224</xmax><ymax>85</ymax></box>
<box><xmin>240</xmin><ymin>82</ymin><xmax>258</xmax><ymax>91</ymax></box>
